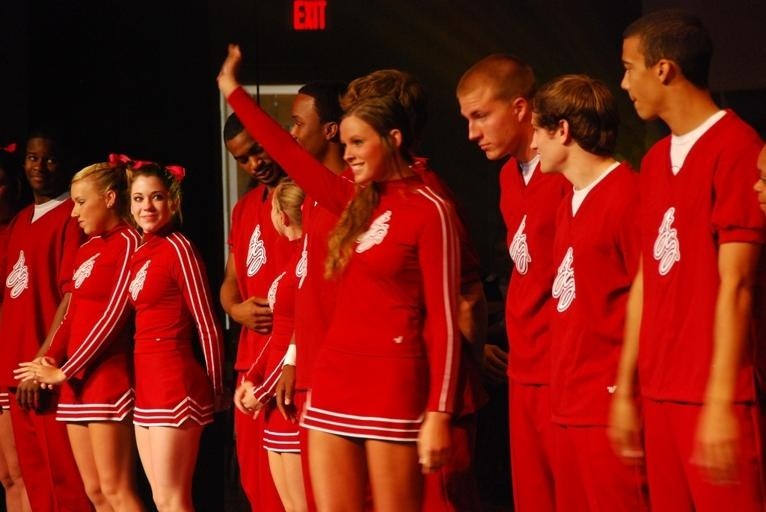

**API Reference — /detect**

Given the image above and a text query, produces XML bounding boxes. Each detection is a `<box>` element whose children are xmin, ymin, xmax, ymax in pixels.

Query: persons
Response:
<box><xmin>121</xmin><ymin>157</ymin><xmax>229</xmax><ymax>511</ymax></box>
<box><xmin>10</xmin><ymin>153</ymin><xmax>152</xmax><ymax>511</ymax></box>
<box><xmin>276</xmin><ymin>74</ymin><xmax>355</xmax><ymax>510</ymax></box>
<box><xmin>606</xmin><ymin>6</ymin><xmax>766</xmax><ymax>512</ymax></box>
<box><xmin>0</xmin><ymin>141</ymin><xmax>31</xmax><ymax>511</ymax></box>
<box><xmin>0</xmin><ymin>120</ymin><xmax>99</xmax><ymax>511</ymax></box>
<box><xmin>454</xmin><ymin>54</ymin><xmax>571</xmax><ymax>512</ymax></box>
<box><xmin>232</xmin><ymin>175</ymin><xmax>311</xmax><ymax>511</ymax></box>
<box><xmin>335</xmin><ymin>66</ymin><xmax>495</xmax><ymax>511</ymax></box>
<box><xmin>217</xmin><ymin>42</ymin><xmax>463</xmax><ymax>511</ymax></box>
<box><xmin>476</xmin><ymin>215</ymin><xmax>518</xmax><ymax>511</ymax></box>
<box><xmin>532</xmin><ymin>71</ymin><xmax>652</xmax><ymax>511</ymax></box>
<box><xmin>219</xmin><ymin>112</ymin><xmax>284</xmax><ymax>511</ymax></box>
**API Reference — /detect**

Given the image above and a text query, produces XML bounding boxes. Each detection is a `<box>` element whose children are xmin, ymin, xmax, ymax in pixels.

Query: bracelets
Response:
<box><xmin>278</xmin><ymin>341</ymin><xmax>299</xmax><ymax>371</ymax></box>
